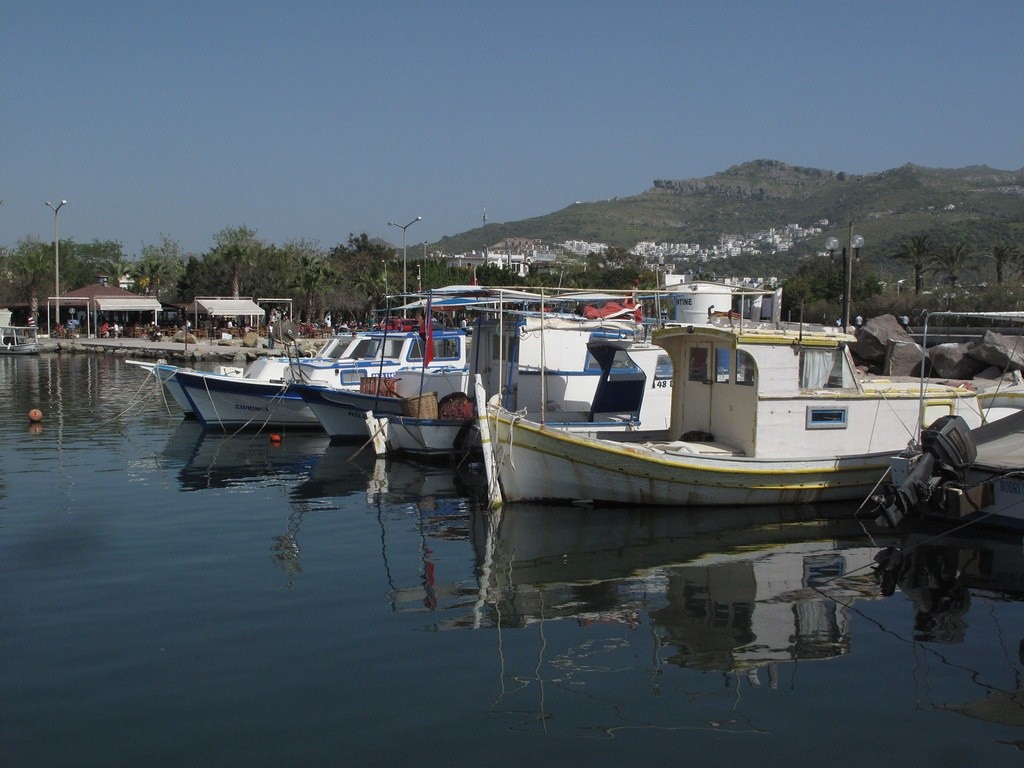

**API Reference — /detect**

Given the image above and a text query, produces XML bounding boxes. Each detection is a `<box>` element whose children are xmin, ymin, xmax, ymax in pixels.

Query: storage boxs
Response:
<box><xmin>360</xmin><ymin>377</ymin><xmax>396</xmax><ymax>397</ymax></box>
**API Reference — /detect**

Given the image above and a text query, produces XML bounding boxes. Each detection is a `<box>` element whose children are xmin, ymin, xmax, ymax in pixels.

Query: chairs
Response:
<box><xmin>130</xmin><ymin>326</ymin><xmax>208</xmax><ymax>339</ymax></box>
<box><xmin>55</xmin><ymin>325</ymin><xmax>82</xmax><ymax>339</ymax></box>
<box><xmin>306</xmin><ymin>327</ymin><xmax>333</xmax><ymax>338</ymax></box>
<box><xmin>97</xmin><ymin>327</ymin><xmax>124</xmax><ymax>338</ymax></box>
<box><xmin>231</xmin><ymin>326</ymin><xmax>266</xmax><ymax>339</ymax></box>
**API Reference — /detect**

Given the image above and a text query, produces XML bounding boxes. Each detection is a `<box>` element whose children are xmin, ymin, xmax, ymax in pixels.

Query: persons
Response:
<box><xmin>392</xmin><ymin>316</ymin><xmax>403</xmax><ymax>332</ymax></box>
<box><xmin>98</xmin><ymin>321</ymin><xmax>148</xmax><ymax>339</ymax></box>
<box><xmin>477</xmin><ymin>312</ymin><xmax>494</xmax><ymax>321</ymax></box>
<box><xmin>50</xmin><ymin>315</ymin><xmax>87</xmax><ymax>338</ymax></box>
<box><xmin>28</xmin><ymin>314</ymin><xmax>35</xmax><ymax>338</ymax></box>
<box><xmin>198</xmin><ymin>315</ymin><xmax>263</xmax><ymax>338</ymax></box>
<box><xmin>145</xmin><ymin>323</ymin><xmax>161</xmax><ymax>342</ymax></box>
<box><xmin>269</xmin><ymin>307</ymin><xmax>332</xmax><ymax>338</ymax></box>
<box><xmin>899</xmin><ymin>314</ymin><xmax>909</xmax><ymax>331</ymax></box>
<box><xmin>334</xmin><ymin>320</ymin><xmax>364</xmax><ymax>334</ymax></box>
<box><xmin>369</xmin><ymin>315</ymin><xmax>374</xmax><ymax>329</ymax></box>
<box><xmin>645</xmin><ymin>298</ymin><xmax>656</xmax><ymax>317</ymax></box>
<box><xmin>415</xmin><ymin>310</ymin><xmax>471</xmax><ymax>328</ymax></box>
<box><xmin>573</xmin><ymin>301</ymin><xmax>603</xmax><ymax>318</ymax></box>
<box><xmin>855</xmin><ymin>311</ymin><xmax>866</xmax><ymax>329</ymax></box>
<box><xmin>165</xmin><ymin>318</ymin><xmax>193</xmax><ymax>336</ymax></box>
<box><xmin>836</xmin><ymin>315</ymin><xmax>842</xmax><ymax>327</ymax></box>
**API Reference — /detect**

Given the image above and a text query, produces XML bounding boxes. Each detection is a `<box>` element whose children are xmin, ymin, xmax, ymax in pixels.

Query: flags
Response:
<box><xmin>419</xmin><ymin>300</ymin><xmax>435</xmax><ymax>367</ymax></box>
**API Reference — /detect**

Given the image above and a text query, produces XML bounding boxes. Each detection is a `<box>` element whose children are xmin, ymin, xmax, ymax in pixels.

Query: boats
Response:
<box><xmin>0</xmin><ymin>326</ymin><xmax>45</xmax><ymax>354</ymax></box>
<box><xmin>125</xmin><ymin>281</ymin><xmax>1024</xmax><ymax>506</ymax></box>
<box><xmin>158</xmin><ymin>420</ymin><xmax>1024</xmax><ymax>691</ymax></box>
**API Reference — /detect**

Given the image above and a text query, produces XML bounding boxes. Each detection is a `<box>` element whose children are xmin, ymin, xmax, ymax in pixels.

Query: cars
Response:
<box><xmin>371</xmin><ymin>316</ymin><xmax>420</xmax><ymax>332</ymax></box>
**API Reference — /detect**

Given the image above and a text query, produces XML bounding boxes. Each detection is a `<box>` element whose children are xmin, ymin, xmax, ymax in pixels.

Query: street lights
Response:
<box><xmin>387</xmin><ymin>216</ymin><xmax>423</xmax><ymax>317</ymax></box>
<box><xmin>207</xmin><ymin>308</ymin><xmax>214</xmax><ymax>346</ymax></box>
<box><xmin>45</xmin><ymin>199</ymin><xmax>68</xmax><ymax>323</ymax></box>
<box><xmin>825</xmin><ymin>235</ymin><xmax>872</xmax><ymax>334</ymax></box>
